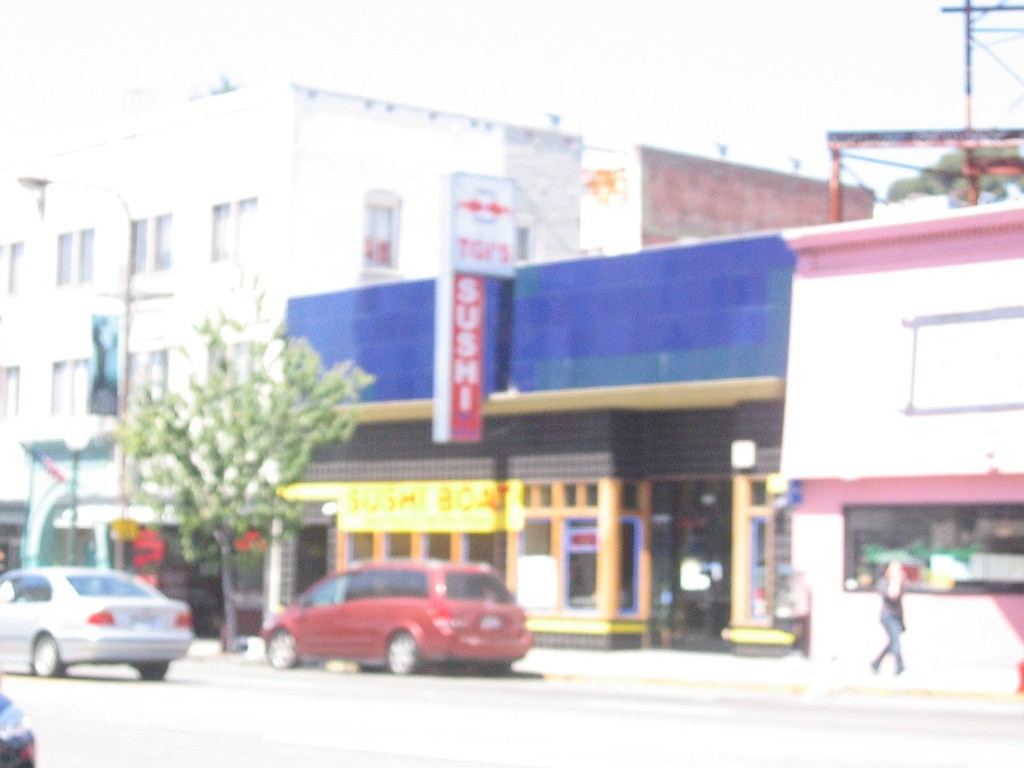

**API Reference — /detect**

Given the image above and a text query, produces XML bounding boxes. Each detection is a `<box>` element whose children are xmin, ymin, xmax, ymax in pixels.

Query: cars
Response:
<box><xmin>260</xmin><ymin>557</ymin><xmax>532</xmax><ymax>675</ymax></box>
<box><xmin>0</xmin><ymin>564</ymin><xmax>194</xmax><ymax>681</ymax></box>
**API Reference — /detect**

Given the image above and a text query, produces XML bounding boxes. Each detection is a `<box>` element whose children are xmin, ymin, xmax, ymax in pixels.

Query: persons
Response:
<box><xmin>869</xmin><ymin>560</ymin><xmax>911</xmax><ymax>678</ymax></box>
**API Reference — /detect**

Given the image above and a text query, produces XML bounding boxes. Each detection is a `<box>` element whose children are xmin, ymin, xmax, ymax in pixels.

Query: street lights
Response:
<box><xmin>17</xmin><ymin>173</ymin><xmax>136</xmax><ymax>571</ymax></box>
<box><xmin>65</xmin><ymin>421</ymin><xmax>91</xmax><ymax>565</ymax></box>
